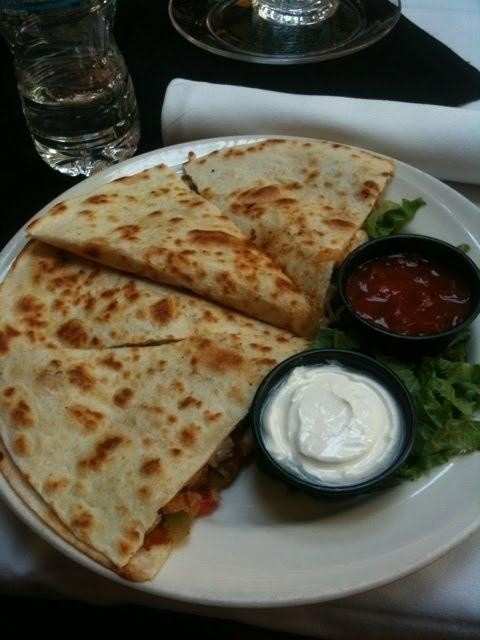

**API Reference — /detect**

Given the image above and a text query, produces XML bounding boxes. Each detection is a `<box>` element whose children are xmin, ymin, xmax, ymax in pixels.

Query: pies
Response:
<box><xmin>1</xmin><ymin>138</ymin><xmax>396</xmax><ymax>582</ymax></box>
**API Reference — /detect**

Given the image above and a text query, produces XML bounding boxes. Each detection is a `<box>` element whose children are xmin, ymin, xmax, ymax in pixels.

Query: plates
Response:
<box><xmin>167</xmin><ymin>0</ymin><xmax>403</xmax><ymax>64</ymax></box>
<box><xmin>1</xmin><ymin>134</ymin><xmax>479</xmax><ymax>610</ymax></box>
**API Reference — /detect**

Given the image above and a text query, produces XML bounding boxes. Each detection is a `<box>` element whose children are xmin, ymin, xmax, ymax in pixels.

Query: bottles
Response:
<box><xmin>1</xmin><ymin>0</ymin><xmax>139</xmax><ymax>177</ymax></box>
